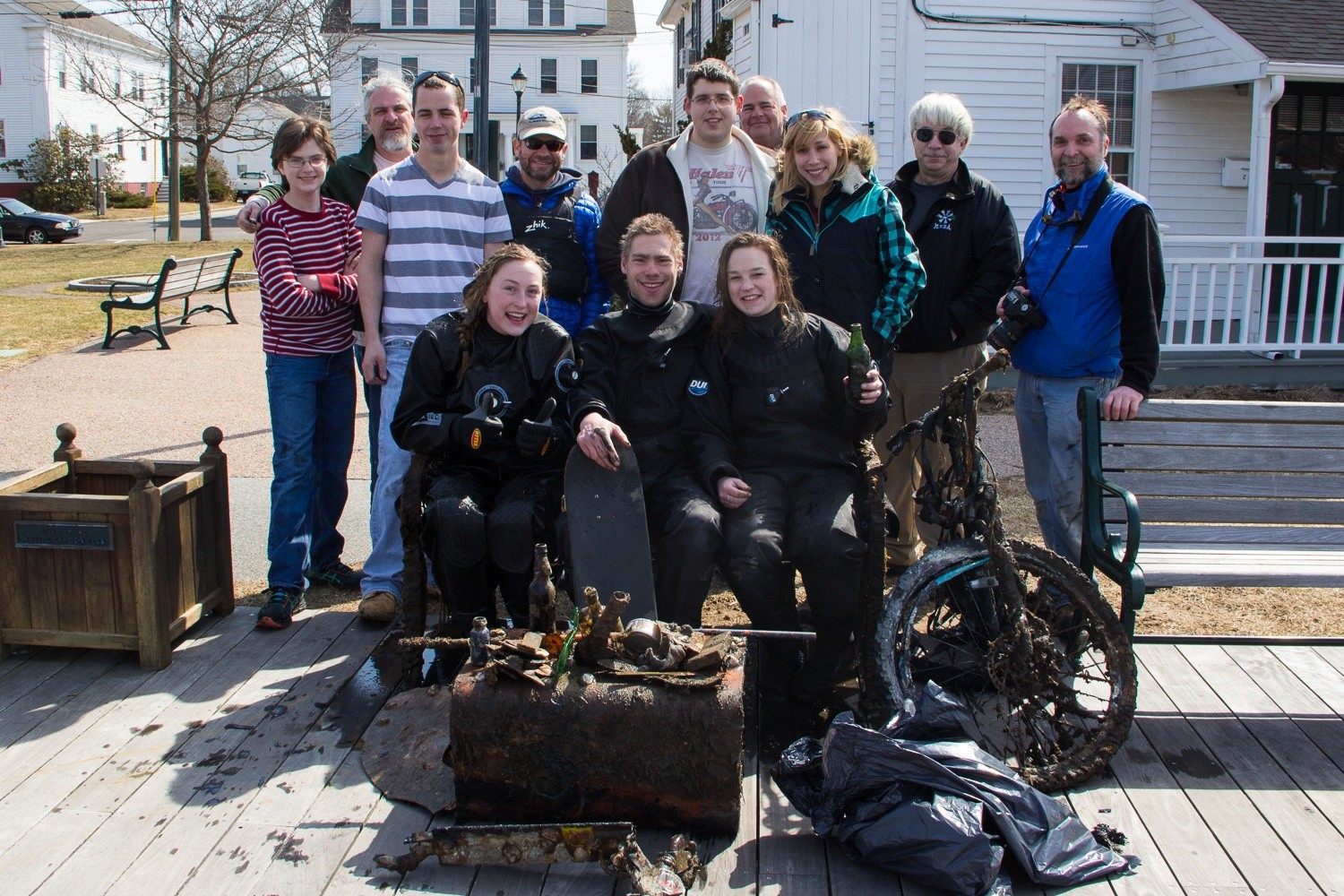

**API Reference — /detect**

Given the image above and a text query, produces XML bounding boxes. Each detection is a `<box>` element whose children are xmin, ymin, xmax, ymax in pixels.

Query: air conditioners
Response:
<box><xmin>678</xmin><ymin>47</ymin><xmax>696</xmax><ymax>69</ymax></box>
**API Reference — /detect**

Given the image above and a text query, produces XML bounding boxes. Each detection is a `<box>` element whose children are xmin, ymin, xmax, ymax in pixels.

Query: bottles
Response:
<box><xmin>470</xmin><ymin>616</ymin><xmax>492</xmax><ymax>667</ymax></box>
<box><xmin>577</xmin><ymin>588</ymin><xmax>624</xmax><ymax>637</ymax></box>
<box><xmin>529</xmin><ymin>543</ymin><xmax>556</xmax><ymax>634</ymax></box>
<box><xmin>846</xmin><ymin>323</ymin><xmax>872</xmax><ymax>401</ymax></box>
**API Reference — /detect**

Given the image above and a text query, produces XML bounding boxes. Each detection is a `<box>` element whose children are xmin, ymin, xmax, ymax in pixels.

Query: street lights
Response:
<box><xmin>511</xmin><ymin>62</ymin><xmax>529</xmax><ymax>137</ymax></box>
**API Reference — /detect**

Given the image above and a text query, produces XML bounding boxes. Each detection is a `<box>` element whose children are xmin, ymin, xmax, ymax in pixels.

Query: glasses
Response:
<box><xmin>525</xmin><ymin>136</ymin><xmax>566</xmax><ymax>151</ymax></box>
<box><xmin>412</xmin><ymin>71</ymin><xmax>464</xmax><ymax>96</ymax></box>
<box><xmin>1041</xmin><ymin>209</ymin><xmax>1081</xmax><ymax>228</ymax></box>
<box><xmin>914</xmin><ymin>127</ymin><xmax>959</xmax><ymax>144</ymax></box>
<box><xmin>282</xmin><ymin>156</ymin><xmax>326</xmax><ymax>168</ymax></box>
<box><xmin>785</xmin><ymin>110</ymin><xmax>835</xmax><ymax>133</ymax></box>
<box><xmin>689</xmin><ymin>95</ymin><xmax>735</xmax><ymax>107</ymax></box>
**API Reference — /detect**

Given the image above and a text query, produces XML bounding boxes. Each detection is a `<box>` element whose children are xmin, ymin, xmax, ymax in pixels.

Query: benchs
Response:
<box><xmin>100</xmin><ymin>247</ymin><xmax>243</xmax><ymax>351</ymax></box>
<box><xmin>1060</xmin><ymin>382</ymin><xmax>1344</xmax><ymax>673</ymax></box>
<box><xmin>394</xmin><ymin>434</ymin><xmax>888</xmax><ymax>689</ymax></box>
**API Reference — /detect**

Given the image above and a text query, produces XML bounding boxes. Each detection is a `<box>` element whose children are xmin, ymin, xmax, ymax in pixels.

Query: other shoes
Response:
<box><xmin>886</xmin><ymin>566</ymin><xmax>908</xmax><ymax>576</ymax></box>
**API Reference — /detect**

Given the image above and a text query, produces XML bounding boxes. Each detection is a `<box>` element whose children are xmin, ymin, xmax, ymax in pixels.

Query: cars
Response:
<box><xmin>0</xmin><ymin>198</ymin><xmax>83</xmax><ymax>245</ymax></box>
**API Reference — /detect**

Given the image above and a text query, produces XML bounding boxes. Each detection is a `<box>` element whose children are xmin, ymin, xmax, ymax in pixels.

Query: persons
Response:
<box><xmin>352</xmin><ymin>68</ymin><xmax>513</xmax><ymax>626</ymax></box>
<box><xmin>387</xmin><ymin>243</ymin><xmax>577</xmax><ymax>634</ymax></box>
<box><xmin>766</xmin><ymin>104</ymin><xmax>927</xmax><ymax>391</ymax></box>
<box><xmin>237</xmin><ymin>56</ymin><xmax>1023</xmax><ymax>632</ymax></box>
<box><xmin>994</xmin><ymin>90</ymin><xmax>1165</xmax><ymax>623</ymax></box>
<box><xmin>687</xmin><ymin>230</ymin><xmax>895</xmax><ymax>666</ymax></box>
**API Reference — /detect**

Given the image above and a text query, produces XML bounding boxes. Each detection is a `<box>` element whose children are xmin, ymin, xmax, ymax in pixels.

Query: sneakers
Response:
<box><xmin>306</xmin><ymin>557</ymin><xmax>360</xmax><ymax>587</ymax></box>
<box><xmin>358</xmin><ymin>590</ymin><xmax>397</xmax><ymax>621</ymax></box>
<box><xmin>257</xmin><ymin>586</ymin><xmax>307</xmax><ymax>628</ymax></box>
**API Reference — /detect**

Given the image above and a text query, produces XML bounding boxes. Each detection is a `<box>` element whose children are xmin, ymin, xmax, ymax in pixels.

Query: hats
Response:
<box><xmin>517</xmin><ymin>106</ymin><xmax>567</xmax><ymax>139</ymax></box>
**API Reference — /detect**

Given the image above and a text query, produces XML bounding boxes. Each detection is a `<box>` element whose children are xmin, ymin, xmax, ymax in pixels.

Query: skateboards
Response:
<box><xmin>561</xmin><ymin>437</ymin><xmax>658</xmax><ymax>633</ymax></box>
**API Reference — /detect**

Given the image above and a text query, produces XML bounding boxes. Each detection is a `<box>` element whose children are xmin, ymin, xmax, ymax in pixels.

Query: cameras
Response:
<box><xmin>986</xmin><ymin>290</ymin><xmax>1044</xmax><ymax>352</ymax></box>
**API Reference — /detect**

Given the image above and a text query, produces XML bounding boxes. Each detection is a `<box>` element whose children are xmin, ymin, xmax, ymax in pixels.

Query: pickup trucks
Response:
<box><xmin>234</xmin><ymin>171</ymin><xmax>276</xmax><ymax>203</ymax></box>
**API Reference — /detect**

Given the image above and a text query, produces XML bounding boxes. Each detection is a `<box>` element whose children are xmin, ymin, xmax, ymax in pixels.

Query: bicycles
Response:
<box><xmin>866</xmin><ymin>349</ymin><xmax>1139</xmax><ymax>792</ymax></box>
